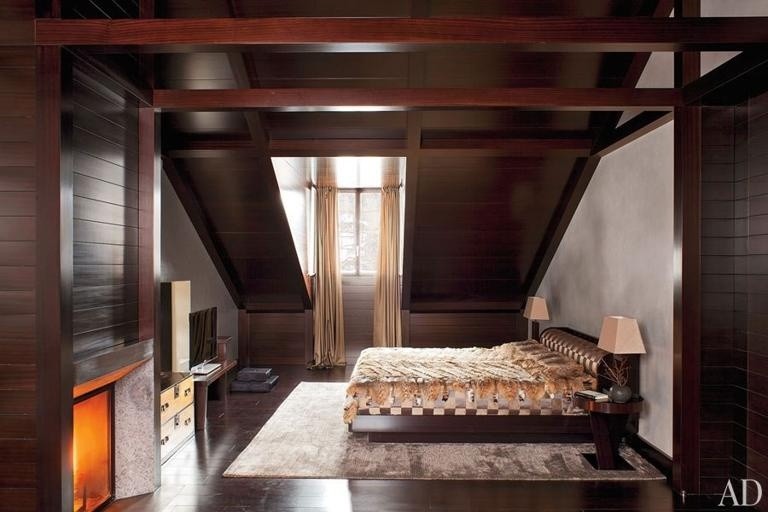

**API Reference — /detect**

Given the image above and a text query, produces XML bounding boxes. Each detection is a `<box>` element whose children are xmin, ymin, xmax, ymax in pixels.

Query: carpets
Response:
<box><xmin>223</xmin><ymin>380</ymin><xmax>667</xmax><ymax>480</ymax></box>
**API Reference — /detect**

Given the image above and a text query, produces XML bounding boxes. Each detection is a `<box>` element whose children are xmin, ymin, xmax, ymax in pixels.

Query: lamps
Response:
<box><xmin>523</xmin><ymin>296</ymin><xmax>550</xmax><ymax>341</ymax></box>
<box><xmin>597</xmin><ymin>315</ymin><xmax>649</xmax><ymax>402</ymax></box>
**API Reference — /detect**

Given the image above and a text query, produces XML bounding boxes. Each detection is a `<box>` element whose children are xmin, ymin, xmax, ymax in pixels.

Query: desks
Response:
<box><xmin>192</xmin><ymin>357</ymin><xmax>239</xmax><ymax>431</ymax></box>
<box><xmin>572</xmin><ymin>390</ymin><xmax>645</xmax><ymax>470</ymax></box>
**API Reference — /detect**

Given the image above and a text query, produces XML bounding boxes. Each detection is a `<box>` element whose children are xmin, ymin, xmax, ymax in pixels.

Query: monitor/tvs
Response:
<box><xmin>189</xmin><ymin>307</ymin><xmax>221</xmax><ymax>374</ymax></box>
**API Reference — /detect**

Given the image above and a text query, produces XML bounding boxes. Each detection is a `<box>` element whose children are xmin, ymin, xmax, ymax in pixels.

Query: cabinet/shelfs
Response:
<box><xmin>159</xmin><ymin>367</ymin><xmax>197</xmax><ymax>465</ymax></box>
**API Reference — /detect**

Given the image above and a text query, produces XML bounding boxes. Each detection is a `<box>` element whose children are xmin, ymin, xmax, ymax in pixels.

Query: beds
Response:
<box><xmin>345</xmin><ymin>328</ymin><xmax>638</xmax><ymax>447</ymax></box>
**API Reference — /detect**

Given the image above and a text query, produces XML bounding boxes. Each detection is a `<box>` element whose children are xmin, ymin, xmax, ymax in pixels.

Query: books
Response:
<box><xmin>574</xmin><ymin>389</ymin><xmax>608</xmax><ymax>402</ymax></box>
